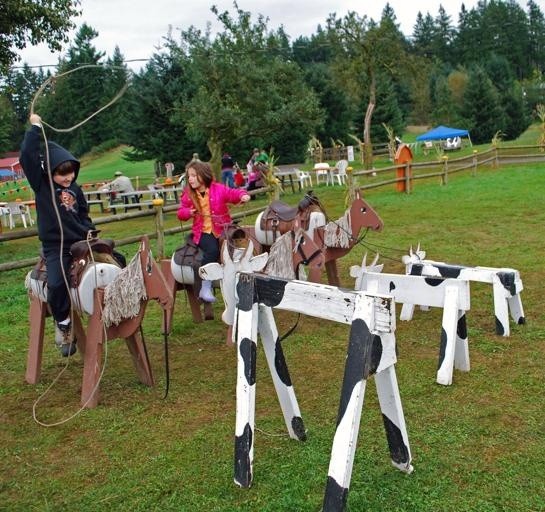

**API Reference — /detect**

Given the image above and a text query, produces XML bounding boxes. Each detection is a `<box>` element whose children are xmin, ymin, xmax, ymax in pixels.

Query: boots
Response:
<box><xmin>57</xmin><ymin>321</ymin><xmax>78</xmax><ymax>356</ymax></box>
<box><xmin>198</xmin><ymin>280</ymin><xmax>217</xmax><ymax>303</ymax></box>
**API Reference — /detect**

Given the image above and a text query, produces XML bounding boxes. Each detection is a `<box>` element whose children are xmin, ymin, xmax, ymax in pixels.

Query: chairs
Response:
<box><xmin>292</xmin><ymin>159</ymin><xmax>349</xmax><ymax>189</ymax></box>
<box><xmin>0</xmin><ymin>203</ymin><xmax>31</xmax><ymax>230</ymax></box>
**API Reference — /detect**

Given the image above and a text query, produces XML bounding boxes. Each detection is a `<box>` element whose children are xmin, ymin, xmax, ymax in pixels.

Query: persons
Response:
<box><xmin>18</xmin><ymin>113</ymin><xmax>125</xmax><ymax>357</ymax></box>
<box><xmin>177</xmin><ymin>162</ymin><xmax>255</xmax><ymax>305</ymax></box>
<box><xmin>96</xmin><ymin>171</ymin><xmax>135</xmax><ymax>213</ymax></box>
<box><xmin>246</xmin><ymin>149</ymin><xmax>269</xmax><ymax>165</ymax></box>
<box><xmin>221</xmin><ymin>150</ymin><xmax>236</xmax><ymax>188</ymax></box>
<box><xmin>245</xmin><ymin>160</ymin><xmax>270</xmax><ymax>201</ymax></box>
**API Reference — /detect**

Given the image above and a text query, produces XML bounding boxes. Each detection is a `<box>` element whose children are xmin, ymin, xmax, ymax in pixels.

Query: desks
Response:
<box><xmin>82</xmin><ymin>188</ymin><xmax>184</xmax><ymax>212</ymax></box>
